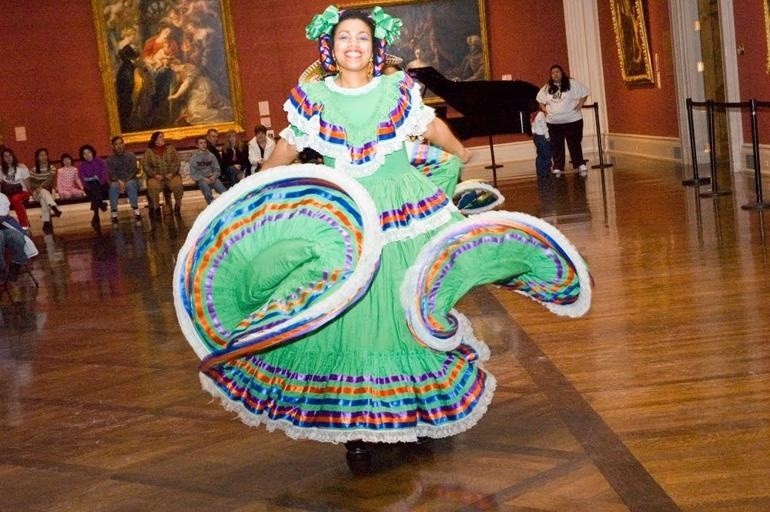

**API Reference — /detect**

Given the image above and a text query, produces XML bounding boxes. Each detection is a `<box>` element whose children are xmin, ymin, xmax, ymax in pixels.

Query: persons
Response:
<box><xmin>1</xmin><ymin>144</ymin><xmax>62</xmax><ymax>284</ymax></box>
<box><xmin>527</xmin><ymin>99</ymin><xmax>554</xmax><ymax>185</ymax></box>
<box><xmin>388</xmin><ymin>14</ymin><xmax>484</xmax><ymax>82</ymax></box>
<box><xmin>190</xmin><ymin>124</ymin><xmax>314</xmax><ymax>205</ymax></box>
<box><xmin>104</xmin><ymin>136</ymin><xmax>142</xmax><ymax>224</ymax></box>
<box><xmin>78</xmin><ymin>144</ymin><xmax>111</xmax><ymax>226</ymax></box>
<box><xmin>102</xmin><ymin>0</ymin><xmax>234</xmax><ymax>134</ymax></box>
<box><xmin>171</xmin><ymin>4</ymin><xmax>595</xmax><ymax>473</ymax></box>
<box><xmin>143</xmin><ymin>131</ymin><xmax>184</xmax><ymax>220</ymax></box>
<box><xmin>535</xmin><ymin>64</ymin><xmax>591</xmax><ymax>173</ymax></box>
<box><xmin>56</xmin><ymin>154</ymin><xmax>86</xmax><ymax>200</ymax></box>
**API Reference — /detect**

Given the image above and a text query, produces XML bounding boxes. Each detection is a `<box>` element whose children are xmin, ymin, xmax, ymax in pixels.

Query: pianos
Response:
<box><xmin>408</xmin><ymin>67</ymin><xmax>538</xmax><ymax>139</ymax></box>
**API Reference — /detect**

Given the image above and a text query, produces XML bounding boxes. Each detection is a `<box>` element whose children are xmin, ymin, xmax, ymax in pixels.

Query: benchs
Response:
<box><xmin>11</xmin><ymin>144</ymin><xmax>225</xmax><ymax>210</ymax></box>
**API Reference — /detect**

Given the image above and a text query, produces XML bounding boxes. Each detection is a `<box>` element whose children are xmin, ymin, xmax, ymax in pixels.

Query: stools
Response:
<box><xmin>0</xmin><ymin>243</ymin><xmax>38</xmax><ymax>306</ymax></box>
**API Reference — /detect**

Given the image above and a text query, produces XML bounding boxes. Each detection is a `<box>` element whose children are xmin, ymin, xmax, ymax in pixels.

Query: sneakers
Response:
<box><xmin>133</xmin><ymin>209</ymin><xmax>140</xmax><ymax>216</ymax></box>
<box><xmin>111</xmin><ymin>212</ymin><xmax>118</xmax><ymax>218</ymax></box>
<box><xmin>554</xmin><ymin>169</ymin><xmax>560</xmax><ymax>173</ymax></box>
<box><xmin>579</xmin><ymin>164</ymin><xmax>588</xmax><ymax>171</ymax></box>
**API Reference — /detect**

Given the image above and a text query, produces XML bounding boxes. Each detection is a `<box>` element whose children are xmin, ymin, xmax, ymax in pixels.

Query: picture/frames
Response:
<box><xmin>336</xmin><ymin>0</ymin><xmax>490</xmax><ymax>105</ymax></box>
<box><xmin>609</xmin><ymin>0</ymin><xmax>654</xmax><ymax>86</ymax></box>
<box><xmin>92</xmin><ymin>0</ymin><xmax>247</xmax><ymax>144</ymax></box>
